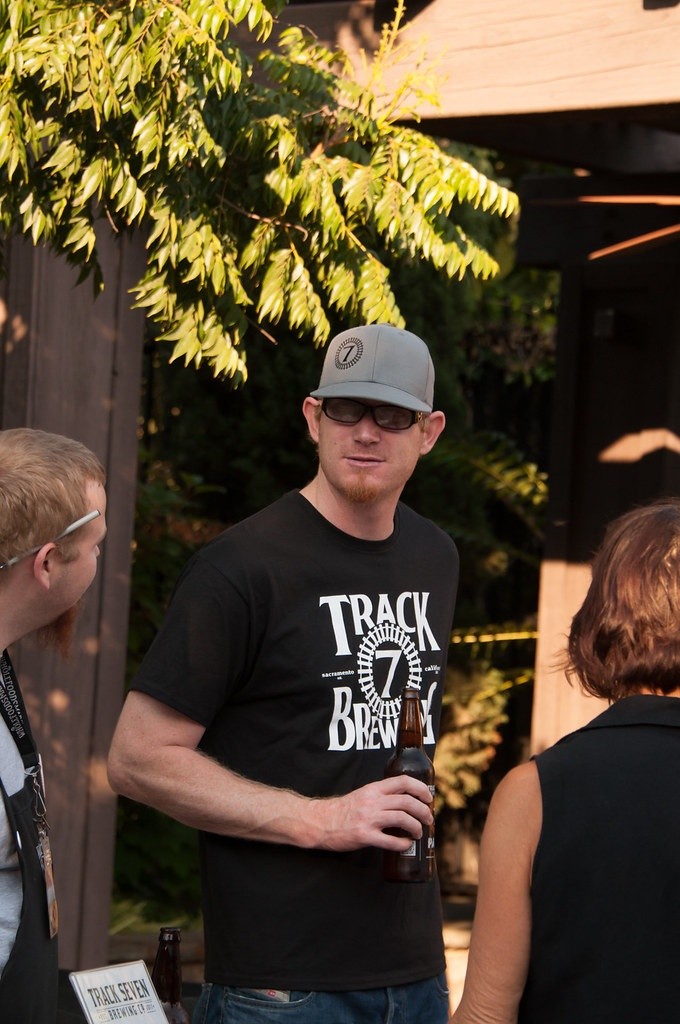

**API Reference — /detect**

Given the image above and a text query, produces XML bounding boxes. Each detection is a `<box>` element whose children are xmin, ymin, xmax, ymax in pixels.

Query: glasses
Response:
<box><xmin>320</xmin><ymin>397</ymin><xmax>426</xmax><ymax>430</ymax></box>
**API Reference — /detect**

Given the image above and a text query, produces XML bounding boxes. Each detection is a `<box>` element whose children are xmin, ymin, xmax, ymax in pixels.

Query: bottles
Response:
<box><xmin>154</xmin><ymin>928</ymin><xmax>188</xmax><ymax>1024</ymax></box>
<box><xmin>383</xmin><ymin>689</ymin><xmax>437</xmax><ymax>881</ymax></box>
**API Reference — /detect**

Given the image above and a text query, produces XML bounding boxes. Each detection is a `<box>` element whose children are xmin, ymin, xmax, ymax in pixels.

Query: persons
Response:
<box><xmin>0</xmin><ymin>426</ymin><xmax>106</xmax><ymax>1024</ymax></box>
<box><xmin>449</xmin><ymin>500</ymin><xmax>679</xmax><ymax>1024</ymax></box>
<box><xmin>107</xmin><ymin>323</ymin><xmax>462</xmax><ymax>1023</ymax></box>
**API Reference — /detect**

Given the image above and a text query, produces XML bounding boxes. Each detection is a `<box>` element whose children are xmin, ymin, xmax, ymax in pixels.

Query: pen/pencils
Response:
<box><xmin>0</xmin><ymin>508</ymin><xmax>101</xmax><ymax>570</ymax></box>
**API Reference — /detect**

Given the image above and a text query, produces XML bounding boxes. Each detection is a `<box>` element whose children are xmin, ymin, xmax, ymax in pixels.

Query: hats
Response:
<box><xmin>310</xmin><ymin>324</ymin><xmax>435</xmax><ymax>413</ymax></box>
